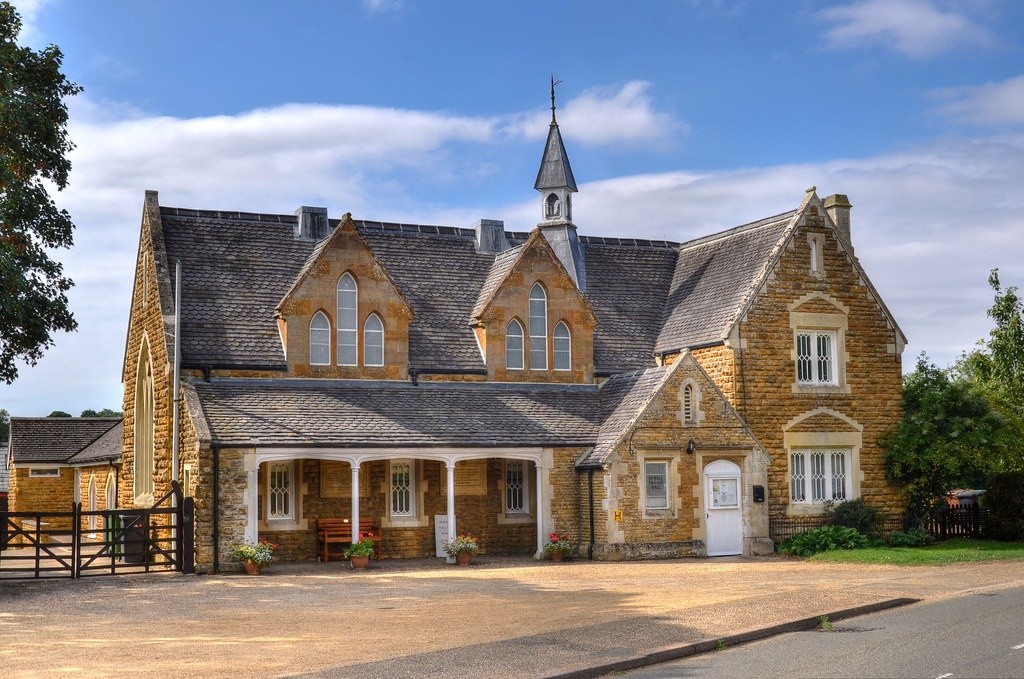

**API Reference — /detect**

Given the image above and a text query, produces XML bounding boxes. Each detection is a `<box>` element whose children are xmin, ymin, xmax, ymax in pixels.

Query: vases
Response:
<box><xmin>455</xmin><ymin>553</ymin><xmax>473</xmax><ymax>564</ymax></box>
<box><xmin>242</xmin><ymin>560</ymin><xmax>262</xmax><ymax>574</ymax></box>
<box><xmin>350</xmin><ymin>556</ymin><xmax>369</xmax><ymax>570</ymax></box>
<box><xmin>552</xmin><ymin>551</ymin><xmax>564</xmax><ymax>562</ymax></box>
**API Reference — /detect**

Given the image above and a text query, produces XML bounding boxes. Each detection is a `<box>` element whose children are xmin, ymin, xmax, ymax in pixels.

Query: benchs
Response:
<box><xmin>317</xmin><ymin>518</ymin><xmax>382</xmax><ymax>563</ymax></box>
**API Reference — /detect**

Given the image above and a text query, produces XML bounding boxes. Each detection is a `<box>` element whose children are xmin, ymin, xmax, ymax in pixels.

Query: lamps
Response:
<box><xmin>686</xmin><ymin>438</ymin><xmax>695</xmax><ymax>454</ymax></box>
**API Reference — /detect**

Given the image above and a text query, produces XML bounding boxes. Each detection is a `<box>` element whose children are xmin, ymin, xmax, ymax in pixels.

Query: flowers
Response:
<box><xmin>542</xmin><ymin>533</ymin><xmax>573</xmax><ymax>557</ymax></box>
<box><xmin>231</xmin><ymin>540</ymin><xmax>277</xmax><ymax>567</ymax></box>
<box><xmin>343</xmin><ymin>532</ymin><xmax>378</xmax><ymax>560</ymax></box>
<box><xmin>442</xmin><ymin>534</ymin><xmax>480</xmax><ymax>558</ymax></box>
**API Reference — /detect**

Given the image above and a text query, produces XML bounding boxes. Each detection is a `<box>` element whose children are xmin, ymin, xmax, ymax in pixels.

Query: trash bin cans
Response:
<box><xmin>102</xmin><ymin>508</ymin><xmax>148</xmax><ymax>564</ymax></box>
